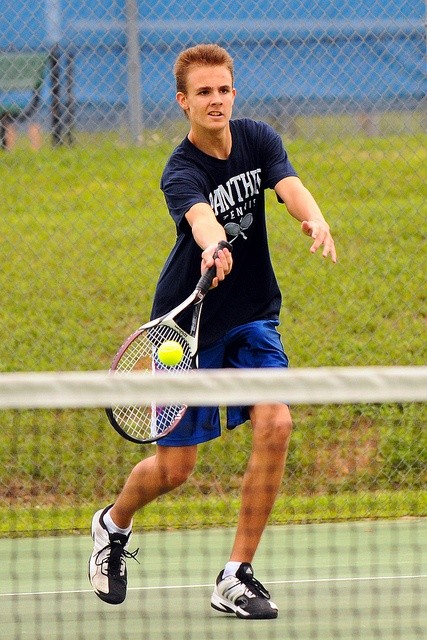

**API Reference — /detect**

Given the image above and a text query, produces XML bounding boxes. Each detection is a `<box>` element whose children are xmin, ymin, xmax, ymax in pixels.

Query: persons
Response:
<box><xmin>89</xmin><ymin>44</ymin><xmax>336</xmax><ymax>620</ymax></box>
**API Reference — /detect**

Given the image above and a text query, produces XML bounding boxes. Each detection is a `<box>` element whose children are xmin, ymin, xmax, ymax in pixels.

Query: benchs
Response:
<box><xmin>0</xmin><ymin>46</ymin><xmax>60</xmax><ymax>148</ymax></box>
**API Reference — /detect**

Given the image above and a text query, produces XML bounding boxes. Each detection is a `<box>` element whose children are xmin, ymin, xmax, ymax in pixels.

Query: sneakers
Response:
<box><xmin>211</xmin><ymin>562</ymin><xmax>280</xmax><ymax>620</ymax></box>
<box><xmin>89</xmin><ymin>503</ymin><xmax>140</xmax><ymax>604</ymax></box>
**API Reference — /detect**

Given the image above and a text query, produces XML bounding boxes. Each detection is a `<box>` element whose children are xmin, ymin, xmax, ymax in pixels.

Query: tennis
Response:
<box><xmin>157</xmin><ymin>340</ymin><xmax>184</xmax><ymax>367</ymax></box>
